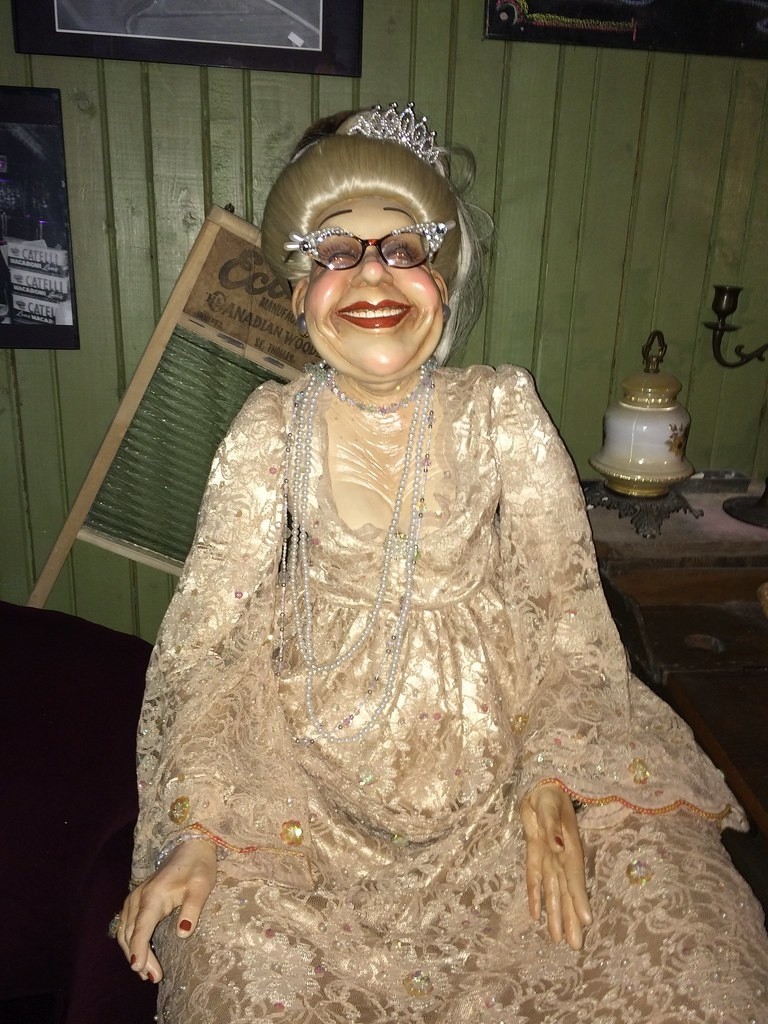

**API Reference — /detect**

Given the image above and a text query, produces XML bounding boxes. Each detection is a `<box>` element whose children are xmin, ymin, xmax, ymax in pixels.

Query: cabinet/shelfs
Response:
<box><xmin>586</xmin><ymin>477</ymin><xmax>767</xmax><ymax>932</ymax></box>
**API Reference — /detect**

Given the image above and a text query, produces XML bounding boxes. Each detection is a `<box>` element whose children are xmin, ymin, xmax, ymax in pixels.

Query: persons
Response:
<box><xmin>115</xmin><ymin>101</ymin><xmax>768</xmax><ymax>1024</ymax></box>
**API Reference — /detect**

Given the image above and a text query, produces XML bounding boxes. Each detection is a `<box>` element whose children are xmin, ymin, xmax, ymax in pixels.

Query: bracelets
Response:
<box><xmin>155</xmin><ymin>832</ymin><xmax>226</xmax><ymax>873</ymax></box>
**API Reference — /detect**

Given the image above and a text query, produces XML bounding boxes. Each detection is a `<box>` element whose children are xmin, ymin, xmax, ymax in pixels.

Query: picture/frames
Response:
<box><xmin>11</xmin><ymin>0</ymin><xmax>363</xmax><ymax>79</ymax></box>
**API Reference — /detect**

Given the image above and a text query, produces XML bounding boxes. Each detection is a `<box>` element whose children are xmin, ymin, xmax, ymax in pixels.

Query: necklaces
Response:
<box><xmin>276</xmin><ymin>356</ymin><xmax>441</xmax><ymax>744</ymax></box>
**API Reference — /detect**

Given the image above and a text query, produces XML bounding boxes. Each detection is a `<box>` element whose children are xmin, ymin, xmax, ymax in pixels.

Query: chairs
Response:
<box><xmin>0</xmin><ymin>604</ymin><xmax>158</xmax><ymax>1024</ymax></box>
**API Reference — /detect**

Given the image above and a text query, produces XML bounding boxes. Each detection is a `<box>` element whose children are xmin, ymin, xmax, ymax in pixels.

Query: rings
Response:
<box><xmin>107</xmin><ymin>914</ymin><xmax>122</xmax><ymax>939</ymax></box>
